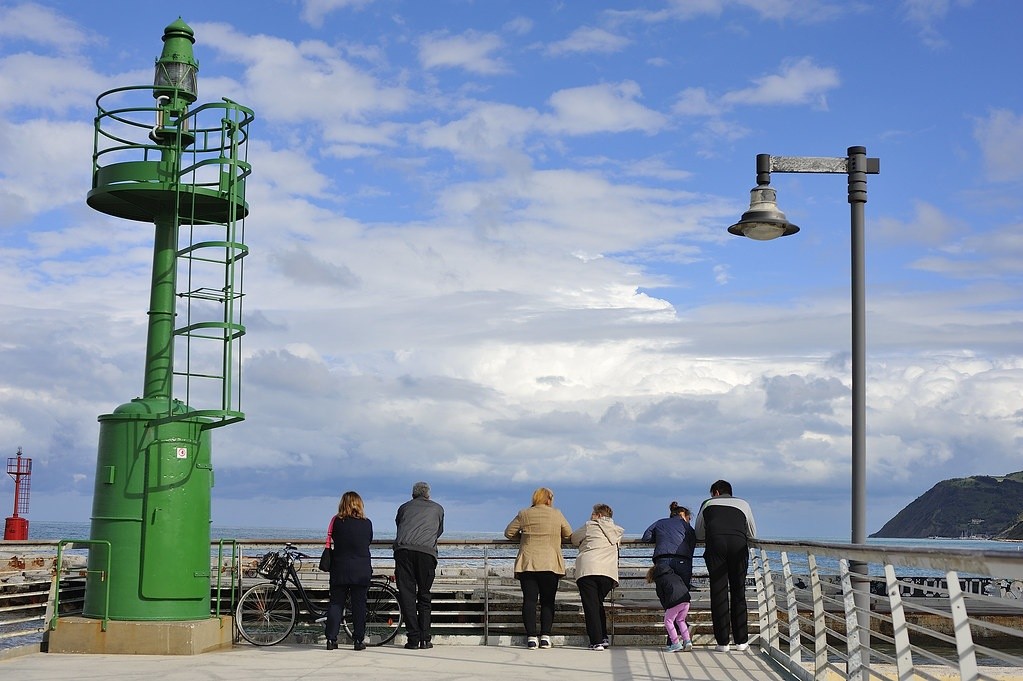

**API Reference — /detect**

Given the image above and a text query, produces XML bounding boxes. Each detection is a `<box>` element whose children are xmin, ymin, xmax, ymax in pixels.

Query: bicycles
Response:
<box><xmin>235</xmin><ymin>543</ymin><xmax>403</xmax><ymax>647</ymax></box>
<box><xmin>235</xmin><ymin>561</ymin><xmax>258</xmax><ymax>578</ymax></box>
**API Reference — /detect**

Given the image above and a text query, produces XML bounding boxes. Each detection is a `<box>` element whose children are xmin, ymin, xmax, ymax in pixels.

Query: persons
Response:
<box><xmin>641</xmin><ymin>501</ymin><xmax>696</xmax><ymax>645</ymax></box>
<box><xmin>695</xmin><ymin>480</ymin><xmax>757</xmax><ymax>652</ymax></box>
<box><xmin>325</xmin><ymin>491</ymin><xmax>374</xmax><ymax>651</ymax></box>
<box><xmin>393</xmin><ymin>481</ymin><xmax>444</xmax><ymax>649</ymax></box>
<box><xmin>646</xmin><ymin>564</ymin><xmax>693</xmax><ymax>651</ymax></box>
<box><xmin>505</xmin><ymin>487</ymin><xmax>573</xmax><ymax>650</ymax></box>
<box><xmin>571</xmin><ymin>503</ymin><xmax>624</xmax><ymax>651</ymax></box>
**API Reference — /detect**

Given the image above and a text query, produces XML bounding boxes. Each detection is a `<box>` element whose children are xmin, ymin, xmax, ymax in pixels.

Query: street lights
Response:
<box><xmin>727</xmin><ymin>146</ymin><xmax>880</xmax><ymax>681</ymax></box>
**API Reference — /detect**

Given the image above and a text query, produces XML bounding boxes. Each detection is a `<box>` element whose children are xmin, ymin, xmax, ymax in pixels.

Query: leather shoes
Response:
<box><xmin>327</xmin><ymin>639</ymin><xmax>338</xmax><ymax>650</ymax></box>
<box><xmin>404</xmin><ymin>643</ymin><xmax>418</xmax><ymax>649</ymax></box>
<box><xmin>354</xmin><ymin>639</ymin><xmax>365</xmax><ymax>650</ymax></box>
<box><xmin>421</xmin><ymin>641</ymin><xmax>433</xmax><ymax>648</ymax></box>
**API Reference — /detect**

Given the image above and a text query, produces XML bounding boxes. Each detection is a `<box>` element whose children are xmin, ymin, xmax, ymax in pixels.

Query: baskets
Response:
<box><xmin>255</xmin><ymin>554</ymin><xmax>287</xmax><ymax>579</ymax></box>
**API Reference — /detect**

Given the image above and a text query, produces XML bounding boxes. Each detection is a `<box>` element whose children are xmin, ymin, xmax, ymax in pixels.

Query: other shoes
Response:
<box><xmin>715</xmin><ymin>644</ymin><xmax>730</xmax><ymax>651</ymax></box>
<box><xmin>736</xmin><ymin>642</ymin><xmax>748</xmax><ymax>650</ymax></box>
<box><xmin>689</xmin><ymin>625</ymin><xmax>695</xmax><ymax>640</ymax></box>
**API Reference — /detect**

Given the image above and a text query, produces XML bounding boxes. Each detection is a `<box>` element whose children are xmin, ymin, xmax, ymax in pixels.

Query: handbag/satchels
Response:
<box><xmin>318</xmin><ymin>545</ymin><xmax>333</xmax><ymax>572</ymax></box>
<box><xmin>258</xmin><ymin>552</ymin><xmax>283</xmax><ymax>580</ymax></box>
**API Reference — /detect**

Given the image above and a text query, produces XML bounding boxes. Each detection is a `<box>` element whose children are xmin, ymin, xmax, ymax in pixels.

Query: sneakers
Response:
<box><xmin>588</xmin><ymin>644</ymin><xmax>604</xmax><ymax>651</ymax></box>
<box><xmin>602</xmin><ymin>639</ymin><xmax>609</xmax><ymax>648</ymax></box>
<box><xmin>683</xmin><ymin>641</ymin><xmax>692</xmax><ymax>652</ymax></box>
<box><xmin>527</xmin><ymin>636</ymin><xmax>539</xmax><ymax>649</ymax></box>
<box><xmin>667</xmin><ymin>642</ymin><xmax>683</xmax><ymax>652</ymax></box>
<box><xmin>541</xmin><ymin>635</ymin><xmax>553</xmax><ymax>648</ymax></box>
